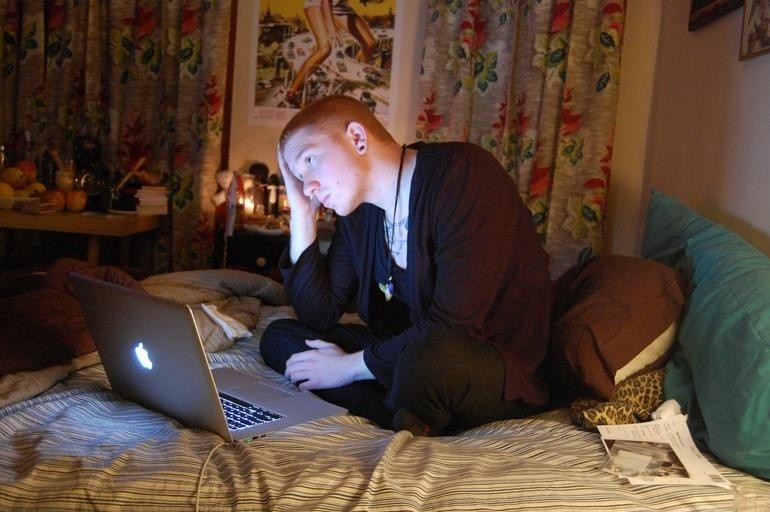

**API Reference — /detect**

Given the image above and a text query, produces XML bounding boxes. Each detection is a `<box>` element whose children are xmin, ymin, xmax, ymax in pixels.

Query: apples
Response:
<box><xmin>0</xmin><ymin>161</ymin><xmax>88</xmax><ymax>212</ymax></box>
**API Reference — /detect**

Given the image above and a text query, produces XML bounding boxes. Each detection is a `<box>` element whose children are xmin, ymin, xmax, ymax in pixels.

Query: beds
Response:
<box><xmin>1</xmin><ymin>186</ymin><xmax>769</xmax><ymax>512</ymax></box>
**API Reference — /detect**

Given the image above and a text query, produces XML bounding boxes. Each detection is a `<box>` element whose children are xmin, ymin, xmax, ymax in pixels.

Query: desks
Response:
<box><xmin>0</xmin><ymin>205</ymin><xmax>168</xmax><ymax>269</ymax></box>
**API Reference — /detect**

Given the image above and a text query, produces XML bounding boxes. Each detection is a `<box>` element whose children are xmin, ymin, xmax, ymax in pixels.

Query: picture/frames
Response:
<box><xmin>687</xmin><ymin>0</ymin><xmax>746</xmax><ymax>33</ymax></box>
<box><xmin>738</xmin><ymin>0</ymin><xmax>770</xmax><ymax>63</ymax></box>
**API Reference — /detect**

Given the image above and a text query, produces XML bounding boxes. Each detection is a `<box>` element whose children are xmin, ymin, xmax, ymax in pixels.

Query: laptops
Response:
<box><xmin>69</xmin><ymin>271</ymin><xmax>350</xmax><ymax>443</ymax></box>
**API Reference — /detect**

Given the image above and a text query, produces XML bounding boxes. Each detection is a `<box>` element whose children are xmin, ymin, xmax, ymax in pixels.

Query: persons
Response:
<box><xmin>282</xmin><ymin>0</ymin><xmax>379</xmax><ymax>109</ymax></box>
<box><xmin>260</xmin><ymin>95</ymin><xmax>554</xmax><ymax>441</ymax></box>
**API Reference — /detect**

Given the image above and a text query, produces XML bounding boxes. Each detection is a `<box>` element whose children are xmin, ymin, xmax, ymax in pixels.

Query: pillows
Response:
<box><xmin>640</xmin><ymin>191</ymin><xmax>770</xmax><ymax>481</ymax></box>
<box><xmin>549</xmin><ymin>253</ymin><xmax>687</xmax><ymax>400</ymax></box>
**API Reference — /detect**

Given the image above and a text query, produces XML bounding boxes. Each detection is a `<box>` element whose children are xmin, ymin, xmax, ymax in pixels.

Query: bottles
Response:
<box><xmin>21</xmin><ymin>131</ymin><xmax>36</xmax><ymax>164</ymax></box>
<box><xmin>41</xmin><ymin>151</ymin><xmax>54</xmax><ymax>184</ymax></box>
<box><xmin>242</xmin><ymin>174</ymin><xmax>279</xmax><ymax>216</ymax></box>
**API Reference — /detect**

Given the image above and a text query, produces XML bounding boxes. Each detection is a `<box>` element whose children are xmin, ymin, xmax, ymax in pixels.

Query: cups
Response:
<box><xmin>56</xmin><ymin>169</ymin><xmax>76</xmax><ymax>190</ymax></box>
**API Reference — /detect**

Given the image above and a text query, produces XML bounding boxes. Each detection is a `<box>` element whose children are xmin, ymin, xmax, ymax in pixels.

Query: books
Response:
<box><xmin>14</xmin><ymin>196</ymin><xmax>56</xmax><ymax>216</ymax></box>
<box><xmin>112</xmin><ymin>181</ymin><xmax>170</xmax><ymax>217</ymax></box>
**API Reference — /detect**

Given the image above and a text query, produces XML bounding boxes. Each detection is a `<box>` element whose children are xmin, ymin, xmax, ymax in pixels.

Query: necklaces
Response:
<box><xmin>379</xmin><ymin>142</ymin><xmax>407</xmax><ymax>302</ymax></box>
